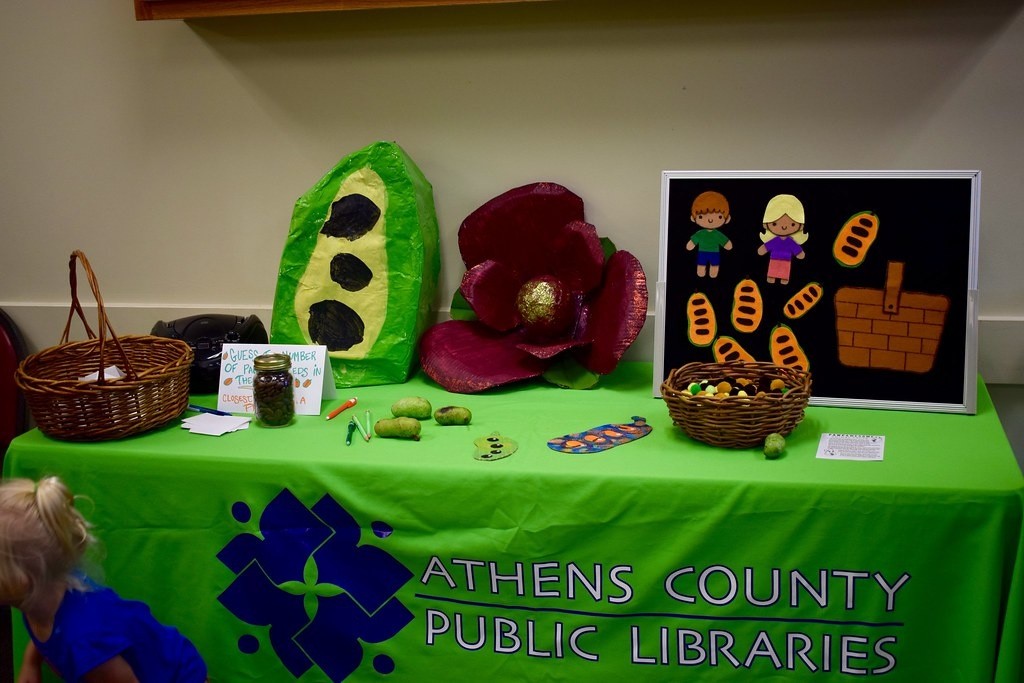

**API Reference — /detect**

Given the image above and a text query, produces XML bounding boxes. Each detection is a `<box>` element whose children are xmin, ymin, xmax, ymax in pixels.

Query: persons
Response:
<box><xmin>0</xmin><ymin>476</ymin><xmax>209</xmax><ymax>683</ymax></box>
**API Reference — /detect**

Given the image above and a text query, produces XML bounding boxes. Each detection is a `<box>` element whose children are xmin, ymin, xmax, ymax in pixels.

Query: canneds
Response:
<box><xmin>252</xmin><ymin>354</ymin><xmax>295</xmax><ymax>426</ymax></box>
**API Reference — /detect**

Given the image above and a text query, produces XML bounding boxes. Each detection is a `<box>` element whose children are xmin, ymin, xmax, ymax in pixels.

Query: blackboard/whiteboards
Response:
<box><xmin>653</xmin><ymin>170</ymin><xmax>981</xmax><ymax>415</ymax></box>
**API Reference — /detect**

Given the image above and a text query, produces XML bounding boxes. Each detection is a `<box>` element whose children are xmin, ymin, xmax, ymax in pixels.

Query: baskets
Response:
<box><xmin>14</xmin><ymin>250</ymin><xmax>194</xmax><ymax>442</ymax></box>
<box><xmin>660</xmin><ymin>361</ymin><xmax>811</xmax><ymax>449</ymax></box>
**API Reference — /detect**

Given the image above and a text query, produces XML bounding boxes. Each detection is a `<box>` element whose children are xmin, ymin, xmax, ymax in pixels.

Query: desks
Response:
<box><xmin>2</xmin><ymin>360</ymin><xmax>1024</xmax><ymax>683</ymax></box>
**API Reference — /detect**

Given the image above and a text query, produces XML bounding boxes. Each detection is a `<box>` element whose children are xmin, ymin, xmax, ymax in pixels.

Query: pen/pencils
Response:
<box><xmin>187</xmin><ymin>404</ymin><xmax>232</xmax><ymax>416</ymax></box>
<box><xmin>366</xmin><ymin>410</ymin><xmax>371</xmax><ymax>439</ymax></box>
<box><xmin>326</xmin><ymin>396</ymin><xmax>357</xmax><ymax>420</ymax></box>
<box><xmin>346</xmin><ymin>421</ymin><xmax>356</xmax><ymax>446</ymax></box>
<box><xmin>352</xmin><ymin>414</ymin><xmax>369</xmax><ymax>441</ymax></box>
